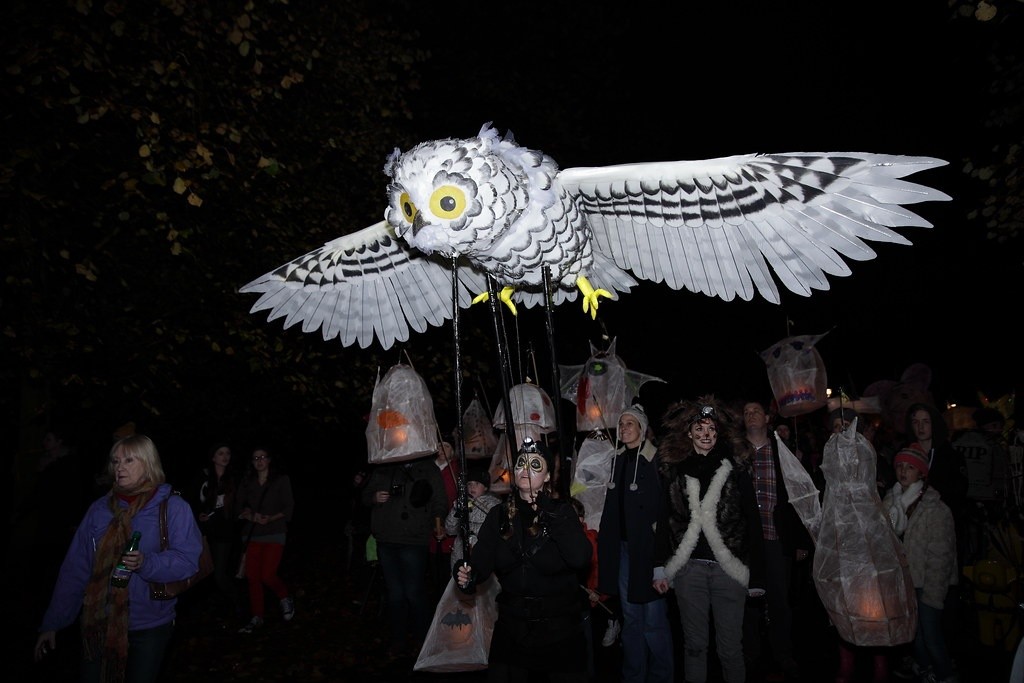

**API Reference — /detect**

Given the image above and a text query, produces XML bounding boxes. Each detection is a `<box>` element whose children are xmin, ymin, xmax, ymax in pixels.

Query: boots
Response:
<box><xmin>835</xmin><ymin>647</ymin><xmax>855</xmax><ymax>683</ymax></box>
<box><xmin>871</xmin><ymin>655</ymin><xmax>890</xmax><ymax>683</ymax></box>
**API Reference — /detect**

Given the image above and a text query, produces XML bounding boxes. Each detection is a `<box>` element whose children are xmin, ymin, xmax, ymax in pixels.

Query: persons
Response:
<box><xmin>196</xmin><ymin>442</ymin><xmax>295</xmax><ymax>634</ymax></box>
<box><xmin>113</xmin><ymin>421</ymin><xmax>136</xmax><ymax>443</ymax></box>
<box><xmin>10</xmin><ymin>427</ymin><xmax>83</xmax><ymax>568</ymax></box>
<box><xmin>34</xmin><ymin>433</ymin><xmax>205</xmax><ymax>683</ymax></box>
<box><xmin>352</xmin><ymin>394</ymin><xmax>1024</xmax><ymax>683</ymax></box>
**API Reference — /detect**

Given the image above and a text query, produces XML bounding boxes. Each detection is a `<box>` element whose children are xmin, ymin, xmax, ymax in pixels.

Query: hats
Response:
<box><xmin>971</xmin><ymin>409</ymin><xmax>1001</xmax><ymax>428</ymax></box>
<box><xmin>828</xmin><ymin>407</ymin><xmax>864</xmax><ymax>434</ymax></box>
<box><xmin>608</xmin><ymin>404</ymin><xmax>649</xmax><ymax>491</ymax></box>
<box><xmin>467</xmin><ymin>464</ymin><xmax>490</xmax><ymax>488</ymax></box>
<box><xmin>894</xmin><ymin>443</ymin><xmax>929</xmax><ymax>478</ymax></box>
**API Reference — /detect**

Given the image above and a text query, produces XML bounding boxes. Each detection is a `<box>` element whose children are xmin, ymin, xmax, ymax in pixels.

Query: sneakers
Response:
<box><xmin>602</xmin><ymin>618</ymin><xmax>621</xmax><ymax>647</ymax></box>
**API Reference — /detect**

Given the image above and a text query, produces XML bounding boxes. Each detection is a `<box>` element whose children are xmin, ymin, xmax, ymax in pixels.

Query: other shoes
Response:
<box><xmin>282</xmin><ymin>602</ymin><xmax>294</xmax><ymax>621</ymax></box>
<box><xmin>745</xmin><ymin>661</ymin><xmax>761</xmax><ymax>672</ymax></box>
<box><xmin>238</xmin><ymin>615</ymin><xmax>265</xmax><ymax>634</ymax></box>
<box><xmin>779</xmin><ymin>656</ymin><xmax>801</xmax><ymax>678</ymax></box>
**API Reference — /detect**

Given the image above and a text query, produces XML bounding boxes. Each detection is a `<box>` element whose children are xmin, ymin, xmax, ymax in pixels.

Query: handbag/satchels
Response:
<box><xmin>149</xmin><ymin>493</ymin><xmax>214</xmax><ymax>601</ymax></box>
<box><xmin>230</xmin><ymin>552</ymin><xmax>247</xmax><ymax>584</ymax></box>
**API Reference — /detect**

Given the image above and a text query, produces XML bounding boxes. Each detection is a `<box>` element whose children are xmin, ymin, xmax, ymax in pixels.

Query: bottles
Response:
<box><xmin>111</xmin><ymin>531</ymin><xmax>142</xmax><ymax>588</ymax></box>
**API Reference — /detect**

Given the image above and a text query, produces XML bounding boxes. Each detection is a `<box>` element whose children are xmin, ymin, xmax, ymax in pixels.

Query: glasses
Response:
<box><xmin>252</xmin><ymin>456</ymin><xmax>265</xmax><ymax>462</ymax></box>
